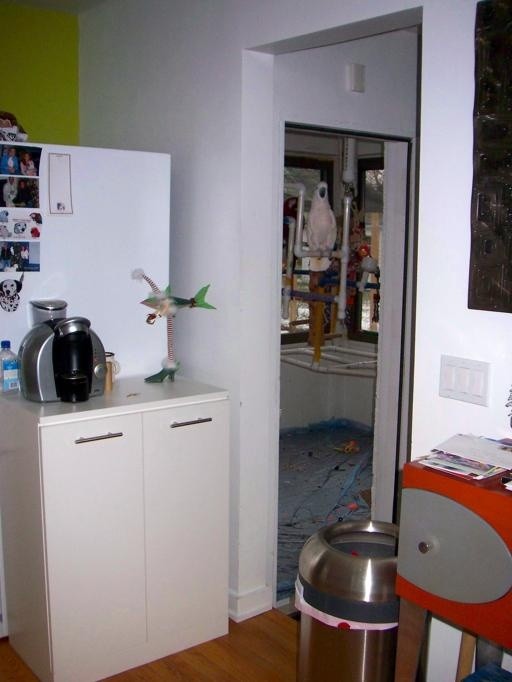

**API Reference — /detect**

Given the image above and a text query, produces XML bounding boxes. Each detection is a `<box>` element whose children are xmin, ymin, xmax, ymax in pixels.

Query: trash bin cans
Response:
<box><xmin>293</xmin><ymin>519</ymin><xmax>400</xmax><ymax>682</ymax></box>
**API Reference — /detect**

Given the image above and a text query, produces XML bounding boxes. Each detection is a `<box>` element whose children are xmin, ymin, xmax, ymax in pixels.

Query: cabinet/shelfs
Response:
<box><xmin>395</xmin><ymin>434</ymin><xmax>512</xmax><ymax>682</ymax></box>
<box><xmin>1</xmin><ymin>373</ymin><xmax>233</xmax><ymax>681</ymax></box>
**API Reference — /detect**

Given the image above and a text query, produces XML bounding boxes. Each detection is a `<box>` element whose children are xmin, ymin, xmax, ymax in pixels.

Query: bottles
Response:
<box><xmin>0</xmin><ymin>339</ymin><xmax>22</xmax><ymax>395</ymax></box>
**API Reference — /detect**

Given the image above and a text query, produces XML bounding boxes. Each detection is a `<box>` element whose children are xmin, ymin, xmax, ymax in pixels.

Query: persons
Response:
<box><xmin>19</xmin><ymin>181</ymin><xmax>33</xmax><ymax>207</ymax></box>
<box><xmin>21</xmin><ymin>150</ymin><xmax>38</xmax><ymax>176</ymax></box>
<box><xmin>3</xmin><ymin>176</ymin><xmax>18</xmax><ymax>207</ymax></box>
<box><xmin>1</xmin><ymin>146</ymin><xmax>23</xmax><ymax>174</ymax></box>
<box><xmin>20</xmin><ymin>243</ymin><xmax>32</xmax><ymax>265</ymax></box>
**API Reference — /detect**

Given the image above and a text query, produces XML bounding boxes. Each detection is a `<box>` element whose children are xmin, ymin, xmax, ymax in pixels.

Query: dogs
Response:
<box><xmin>0</xmin><ymin>278</ymin><xmax>22</xmax><ymax>313</ymax></box>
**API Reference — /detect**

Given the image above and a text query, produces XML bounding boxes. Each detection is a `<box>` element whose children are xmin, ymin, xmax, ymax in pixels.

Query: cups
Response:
<box><xmin>105</xmin><ymin>350</ymin><xmax>121</xmax><ymax>396</ymax></box>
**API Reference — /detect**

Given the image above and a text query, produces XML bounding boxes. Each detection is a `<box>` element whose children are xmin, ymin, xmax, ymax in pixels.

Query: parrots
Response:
<box><xmin>306</xmin><ymin>181</ymin><xmax>338</xmax><ymax>369</ymax></box>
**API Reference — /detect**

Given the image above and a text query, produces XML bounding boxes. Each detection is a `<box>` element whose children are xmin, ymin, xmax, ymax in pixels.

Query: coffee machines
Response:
<box><xmin>15</xmin><ymin>298</ymin><xmax>108</xmax><ymax>405</ymax></box>
